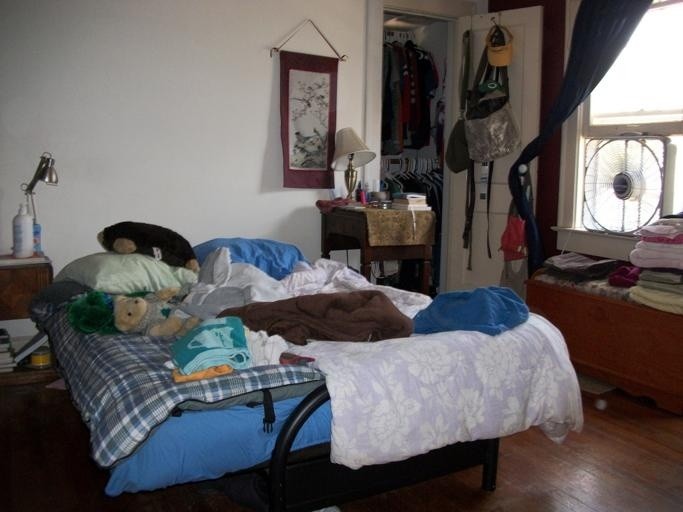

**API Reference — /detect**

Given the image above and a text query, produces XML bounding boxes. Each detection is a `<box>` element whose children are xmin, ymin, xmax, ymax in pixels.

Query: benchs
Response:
<box><xmin>523</xmin><ymin>268</ymin><xmax>683</xmax><ymax>415</ymax></box>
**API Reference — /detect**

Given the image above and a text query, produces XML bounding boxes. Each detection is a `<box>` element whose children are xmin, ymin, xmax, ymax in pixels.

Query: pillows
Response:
<box><xmin>53</xmin><ymin>237</ymin><xmax>301</xmax><ymax>295</ymax></box>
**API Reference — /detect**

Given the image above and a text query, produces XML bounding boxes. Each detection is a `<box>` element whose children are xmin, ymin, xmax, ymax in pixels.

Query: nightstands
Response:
<box><xmin>315</xmin><ymin>199</ymin><xmax>434</xmax><ymax>300</ymax></box>
<box><xmin>0</xmin><ymin>253</ymin><xmax>54</xmax><ymax>407</ymax></box>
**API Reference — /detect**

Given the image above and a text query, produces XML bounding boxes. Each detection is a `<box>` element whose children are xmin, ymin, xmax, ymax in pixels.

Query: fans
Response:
<box><xmin>581</xmin><ymin>134</ymin><xmax>678</xmax><ymax>238</ymax></box>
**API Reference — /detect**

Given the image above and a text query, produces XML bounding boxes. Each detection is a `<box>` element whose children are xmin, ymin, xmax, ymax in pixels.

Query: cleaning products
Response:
<box><xmin>32</xmin><ymin>218</ymin><xmax>41</xmax><ymax>256</ymax></box>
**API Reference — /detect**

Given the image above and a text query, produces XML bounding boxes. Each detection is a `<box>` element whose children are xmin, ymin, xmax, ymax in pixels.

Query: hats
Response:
<box><xmin>485</xmin><ymin>26</ymin><xmax>514</xmax><ymax>67</ymax></box>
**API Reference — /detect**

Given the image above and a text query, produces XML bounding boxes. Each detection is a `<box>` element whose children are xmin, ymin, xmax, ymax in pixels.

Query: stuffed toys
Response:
<box><xmin>62</xmin><ymin>288</ymin><xmax>156</xmax><ymax>336</ymax></box>
<box><xmin>111</xmin><ymin>286</ymin><xmax>200</xmax><ymax>335</ymax></box>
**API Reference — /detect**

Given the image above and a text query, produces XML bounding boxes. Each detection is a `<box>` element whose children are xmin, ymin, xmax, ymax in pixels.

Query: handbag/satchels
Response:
<box><xmin>466</xmin><ymin>80</ymin><xmax>508</xmax><ymax>117</ymax></box>
<box><xmin>465</xmin><ymin>97</ymin><xmax>520</xmax><ymax>162</ymax></box>
<box><xmin>499</xmin><ymin>262</ymin><xmax>528</xmax><ymax>305</ymax></box>
<box><xmin>446</xmin><ymin>120</ymin><xmax>471</xmax><ymax>173</ymax></box>
<box><xmin>501</xmin><ymin>216</ymin><xmax>527</xmax><ymax>261</ymax></box>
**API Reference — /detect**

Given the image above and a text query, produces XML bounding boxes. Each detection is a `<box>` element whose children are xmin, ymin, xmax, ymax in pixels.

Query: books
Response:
<box><xmin>390</xmin><ymin>192</ymin><xmax>431</xmax><ymax>212</ymax></box>
<box><xmin>0</xmin><ymin>317</ymin><xmax>49</xmax><ymax>374</ymax></box>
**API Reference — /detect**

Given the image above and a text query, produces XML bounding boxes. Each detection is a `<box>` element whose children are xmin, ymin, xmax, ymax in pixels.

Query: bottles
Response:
<box><xmin>12</xmin><ymin>205</ymin><xmax>35</xmax><ymax>259</ymax></box>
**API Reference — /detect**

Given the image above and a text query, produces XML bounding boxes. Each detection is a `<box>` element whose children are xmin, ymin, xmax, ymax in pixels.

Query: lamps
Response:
<box><xmin>21</xmin><ymin>153</ymin><xmax>59</xmax><ymax>223</ymax></box>
<box><xmin>328</xmin><ymin>127</ymin><xmax>376</xmax><ymax>205</ymax></box>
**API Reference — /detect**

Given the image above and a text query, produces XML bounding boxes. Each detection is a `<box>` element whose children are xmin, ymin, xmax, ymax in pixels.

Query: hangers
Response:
<box><xmin>382</xmin><ymin>29</ymin><xmax>418</xmax><ymax>54</ymax></box>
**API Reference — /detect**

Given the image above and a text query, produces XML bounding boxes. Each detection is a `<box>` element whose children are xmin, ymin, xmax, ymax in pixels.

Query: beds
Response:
<box><xmin>42</xmin><ymin>266</ymin><xmax>503</xmax><ymax>511</ymax></box>
